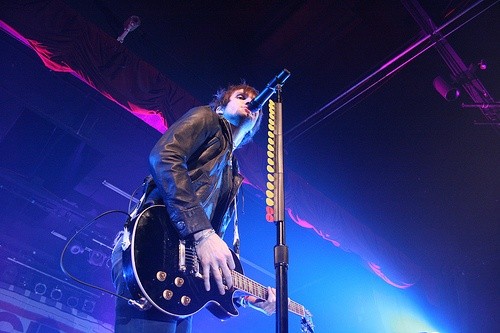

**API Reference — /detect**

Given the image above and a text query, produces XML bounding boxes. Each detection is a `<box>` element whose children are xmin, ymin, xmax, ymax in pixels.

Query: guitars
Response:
<box><xmin>120</xmin><ymin>203</ymin><xmax>315</xmax><ymax>333</ymax></box>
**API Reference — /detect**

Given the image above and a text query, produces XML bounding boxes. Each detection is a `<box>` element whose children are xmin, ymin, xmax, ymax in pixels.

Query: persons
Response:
<box><xmin>110</xmin><ymin>83</ymin><xmax>290</xmax><ymax>333</ymax></box>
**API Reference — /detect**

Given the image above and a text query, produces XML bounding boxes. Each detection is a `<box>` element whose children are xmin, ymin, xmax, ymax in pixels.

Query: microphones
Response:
<box><xmin>248</xmin><ymin>69</ymin><xmax>291</xmax><ymax>112</ymax></box>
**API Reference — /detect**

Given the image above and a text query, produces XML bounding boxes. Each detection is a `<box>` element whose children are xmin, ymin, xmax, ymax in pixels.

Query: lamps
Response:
<box><xmin>117</xmin><ymin>14</ymin><xmax>142</xmax><ymax>45</ymax></box>
<box><xmin>433</xmin><ymin>76</ymin><xmax>463</xmax><ymax>102</ymax></box>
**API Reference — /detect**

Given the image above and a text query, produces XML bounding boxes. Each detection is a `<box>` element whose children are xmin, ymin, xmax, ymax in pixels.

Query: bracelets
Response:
<box><xmin>193</xmin><ymin>229</ymin><xmax>215</xmax><ymax>245</ymax></box>
